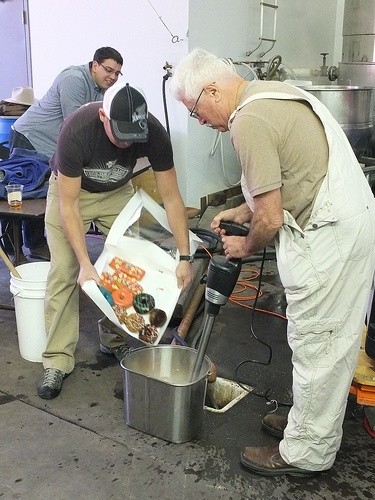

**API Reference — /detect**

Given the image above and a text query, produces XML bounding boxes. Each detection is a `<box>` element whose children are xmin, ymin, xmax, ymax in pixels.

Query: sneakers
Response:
<box><xmin>100</xmin><ymin>342</ymin><xmax>134</xmax><ymax>360</ymax></box>
<box><xmin>37</xmin><ymin>368</ymin><xmax>69</xmax><ymax>399</ymax></box>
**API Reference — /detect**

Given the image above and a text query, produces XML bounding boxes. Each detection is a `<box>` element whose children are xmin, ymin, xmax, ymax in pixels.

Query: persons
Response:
<box><xmin>1</xmin><ymin>47</ymin><xmax>123</xmax><ymax>266</ymax></box>
<box><xmin>170</xmin><ymin>47</ymin><xmax>375</xmax><ymax>475</ymax></box>
<box><xmin>36</xmin><ymin>83</ymin><xmax>194</xmax><ymax>399</ymax></box>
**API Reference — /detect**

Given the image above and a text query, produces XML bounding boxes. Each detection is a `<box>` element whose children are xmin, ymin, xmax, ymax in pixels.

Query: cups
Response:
<box><xmin>5</xmin><ymin>184</ymin><xmax>24</xmax><ymax>207</ymax></box>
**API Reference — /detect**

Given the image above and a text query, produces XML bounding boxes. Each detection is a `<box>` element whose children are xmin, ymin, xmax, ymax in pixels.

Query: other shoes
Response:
<box><xmin>9</xmin><ymin>254</ymin><xmax>29</xmax><ymax>267</ymax></box>
<box><xmin>28</xmin><ymin>245</ymin><xmax>50</xmax><ymax>260</ymax></box>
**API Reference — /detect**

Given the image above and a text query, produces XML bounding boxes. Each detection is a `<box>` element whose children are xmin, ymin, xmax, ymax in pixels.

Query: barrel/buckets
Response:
<box><xmin>9</xmin><ymin>262</ymin><xmax>51</xmax><ymax>363</ymax></box>
<box><xmin>120</xmin><ymin>346</ymin><xmax>212</xmax><ymax>444</ymax></box>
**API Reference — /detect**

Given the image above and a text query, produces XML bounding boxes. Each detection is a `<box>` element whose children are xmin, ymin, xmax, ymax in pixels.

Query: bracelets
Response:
<box><xmin>180</xmin><ymin>255</ymin><xmax>193</xmax><ymax>262</ymax></box>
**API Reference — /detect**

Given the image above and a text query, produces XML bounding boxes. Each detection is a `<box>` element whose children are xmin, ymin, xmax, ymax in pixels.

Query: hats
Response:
<box><xmin>103</xmin><ymin>82</ymin><xmax>148</xmax><ymax>143</ymax></box>
<box><xmin>4</xmin><ymin>87</ymin><xmax>38</xmax><ymax>105</ymax></box>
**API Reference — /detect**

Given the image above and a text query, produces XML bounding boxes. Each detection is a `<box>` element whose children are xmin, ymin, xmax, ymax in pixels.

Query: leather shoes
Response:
<box><xmin>262</xmin><ymin>414</ymin><xmax>287</xmax><ymax>437</ymax></box>
<box><xmin>241</xmin><ymin>444</ymin><xmax>320</xmax><ymax>478</ymax></box>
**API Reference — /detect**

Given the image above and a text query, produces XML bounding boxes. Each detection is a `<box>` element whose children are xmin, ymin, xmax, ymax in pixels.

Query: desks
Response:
<box><xmin>0</xmin><ymin>198</ymin><xmax>46</xmax><ymax>267</ymax></box>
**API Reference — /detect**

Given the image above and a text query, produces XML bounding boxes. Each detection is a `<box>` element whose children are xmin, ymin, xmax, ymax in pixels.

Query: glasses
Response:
<box><xmin>96</xmin><ymin>59</ymin><xmax>123</xmax><ymax>78</ymax></box>
<box><xmin>190</xmin><ymin>82</ymin><xmax>215</xmax><ymax>119</ymax></box>
